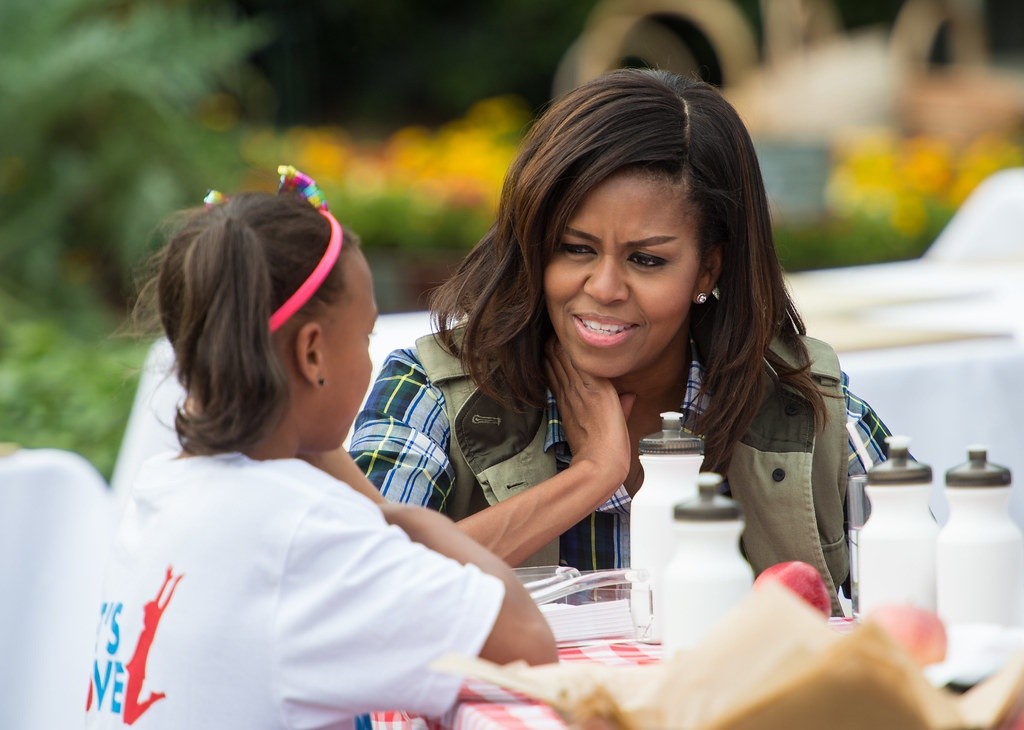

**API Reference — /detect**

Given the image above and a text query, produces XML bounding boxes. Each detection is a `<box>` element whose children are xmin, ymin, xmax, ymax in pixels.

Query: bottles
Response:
<box><xmin>936</xmin><ymin>447</ymin><xmax>1023</xmax><ymax>686</ymax></box>
<box><xmin>655</xmin><ymin>470</ymin><xmax>754</xmax><ymax>650</ymax></box>
<box><xmin>629</xmin><ymin>411</ymin><xmax>706</xmax><ymax>645</ymax></box>
<box><xmin>862</xmin><ymin>434</ymin><xmax>947</xmax><ymax>689</ymax></box>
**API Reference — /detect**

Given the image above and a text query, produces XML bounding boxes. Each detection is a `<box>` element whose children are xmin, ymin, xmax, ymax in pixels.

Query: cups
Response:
<box><xmin>850</xmin><ymin>474</ymin><xmax>868</xmax><ymax>620</ymax></box>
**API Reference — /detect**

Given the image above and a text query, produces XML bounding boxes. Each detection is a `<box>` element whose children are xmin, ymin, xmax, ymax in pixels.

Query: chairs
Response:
<box><xmin>0</xmin><ymin>446</ymin><xmax>108</xmax><ymax>729</ymax></box>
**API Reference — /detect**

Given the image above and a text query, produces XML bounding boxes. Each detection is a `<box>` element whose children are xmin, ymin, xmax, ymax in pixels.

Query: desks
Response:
<box><xmin>104</xmin><ymin>165</ymin><xmax>1023</xmax><ymax>514</ymax></box>
<box><xmin>370</xmin><ymin>637</ymin><xmax>663</xmax><ymax>730</ymax></box>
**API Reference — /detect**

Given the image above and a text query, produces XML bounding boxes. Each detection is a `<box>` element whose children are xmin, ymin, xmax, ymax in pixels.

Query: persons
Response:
<box><xmin>84</xmin><ymin>164</ymin><xmax>560</xmax><ymax>730</ymax></box>
<box><xmin>350</xmin><ymin>66</ymin><xmax>918</xmax><ymax>618</ymax></box>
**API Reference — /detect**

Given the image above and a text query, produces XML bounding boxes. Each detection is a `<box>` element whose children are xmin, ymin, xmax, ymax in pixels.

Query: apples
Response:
<box><xmin>864</xmin><ymin>601</ymin><xmax>947</xmax><ymax>669</ymax></box>
<box><xmin>754</xmin><ymin>561</ymin><xmax>831</xmax><ymax>622</ymax></box>
